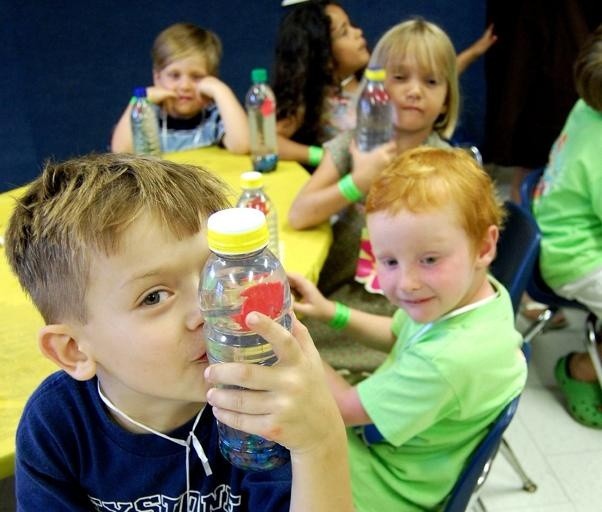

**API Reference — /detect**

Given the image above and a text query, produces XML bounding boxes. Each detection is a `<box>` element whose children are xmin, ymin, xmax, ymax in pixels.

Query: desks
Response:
<box><xmin>0</xmin><ymin>144</ymin><xmax>335</xmax><ymax>477</ymax></box>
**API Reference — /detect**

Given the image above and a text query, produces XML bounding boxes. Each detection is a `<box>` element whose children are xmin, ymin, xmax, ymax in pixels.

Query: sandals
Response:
<box><xmin>553</xmin><ymin>349</ymin><xmax>602</xmax><ymax>430</ymax></box>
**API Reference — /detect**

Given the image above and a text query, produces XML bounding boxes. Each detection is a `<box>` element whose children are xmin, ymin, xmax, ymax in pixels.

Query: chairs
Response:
<box><xmin>446</xmin><ymin>198</ymin><xmax>542</xmax><ymax>512</ymax></box>
<box><xmin>520</xmin><ymin>168</ymin><xmax>602</xmax><ymax>387</ymax></box>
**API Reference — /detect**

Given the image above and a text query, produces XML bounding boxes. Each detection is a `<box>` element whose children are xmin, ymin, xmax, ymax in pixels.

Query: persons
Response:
<box><xmin>110</xmin><ymin>22</ymin><xmax>254</xmax><ymax>156</ymax></box>
<box><xmin>261</xmin><ymin>1</ymin><xmax>498</xmax><ymax>304</ymax></box>
<box><xmin>2</xmin><ymin>149</ymin><xmax>358</xmax><ymax>512</ymax></box>
<box><xmin>285</xmin><ymin>146</ymin><xmax>528</xmax><ymax>512</ymax></box>
<box><xmin>530</xmin><ymin>19</ymin><xmax>602</xmax><ymax>428</ymax></box>
<box><xmin>295</xmin><ymin>17</ymin><xmax>462</xmax><ymax>385</ymax></box>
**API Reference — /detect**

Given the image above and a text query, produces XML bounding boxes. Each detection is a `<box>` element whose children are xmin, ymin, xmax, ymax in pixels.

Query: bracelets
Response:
<box><xmin>129</xmin><ymin>86</ymin><xmax>154</xmax><ymax>108</ymax></box>
<box><xmin>327</xmin><ymin>300</ymin><xmax>351</xmax><ymax>334</ymax></box>
<box><xmin>337</xmin><ymin>173</ymin><xmax>364</xmax><ymax>204</ymax></box>
<box><xmin>308</xmin><ymin>144</ymin><xmax>325</xmax><ymax>168</ymax></box>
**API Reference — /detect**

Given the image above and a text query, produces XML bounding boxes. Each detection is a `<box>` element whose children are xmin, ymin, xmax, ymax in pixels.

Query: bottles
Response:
<box><xmin>356</xmin><ymin>66</ymin><xmax>395</xmax><ymax>153</ymax></box>
<box><xmin>244</xmin><ymin>66</ymin><xmax>279</xmax><ymax>172</ymax></box>
<box><xmin>197</xmin><ymin>205</ymin><xmax>295</xmax><ymax>472</ymax></box>
<box><xmin>235</xmin><ymin>170</ymin><xmax>279</xmax><ymax>265</ymax></box>
<box><xmin>130</xmin><ymin>86</ymin><xmax>162</xmax><ymax>162</ymax></box>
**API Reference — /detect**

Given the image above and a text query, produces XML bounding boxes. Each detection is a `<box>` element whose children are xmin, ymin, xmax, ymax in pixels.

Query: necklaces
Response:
<box><xmin>334</xmin><ymin>73</ymin><xmax>356</xmax><ymax>87</ymax></box>
